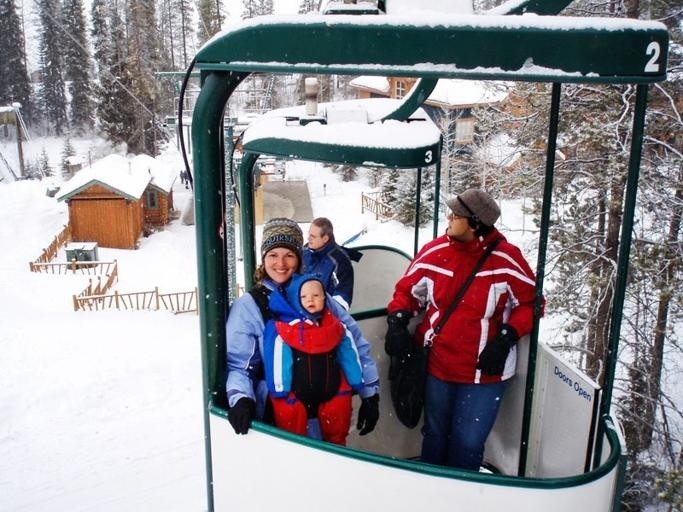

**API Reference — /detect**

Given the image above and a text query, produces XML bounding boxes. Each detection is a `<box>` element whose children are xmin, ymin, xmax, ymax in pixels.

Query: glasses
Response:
<box><xmin>452</xmin><ymin>212</ymin><xmax>471</xmax><ymax>220</ymax></box>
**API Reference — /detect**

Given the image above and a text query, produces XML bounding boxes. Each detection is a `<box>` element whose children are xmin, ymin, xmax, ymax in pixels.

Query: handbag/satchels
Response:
<box><xmin>388</xmin><ymin>347</ymin><xmax>427</xmax><ymax>429</ymax></box>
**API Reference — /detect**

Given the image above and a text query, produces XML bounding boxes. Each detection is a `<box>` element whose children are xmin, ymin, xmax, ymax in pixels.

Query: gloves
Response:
<box><xmin>357</xmin><ymin>394</ymin><xmax>379</xmax><ymax>436</ymax></box>
<box><xmin>385</xmin><ymin>311</ymin><xmax>416</xmax><ymax>354</ymax></box>
<box><xmin>228</xmin><ymin>398</ymin><xmax>256</xmax><ymax>434</ymax></box>
<box><xmin>476</xmin><ymin>323</ymin><xmax>520</xmax><ymax>376</ymax></box>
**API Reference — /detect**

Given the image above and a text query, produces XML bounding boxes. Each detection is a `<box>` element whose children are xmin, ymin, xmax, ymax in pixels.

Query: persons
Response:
<box><xmin>384</xmin><ymin>188</ymin><xmax>545</xmax><ymax>470</ymax></box>
<box><xmin>223</xmin><ymin>216</ymin><xmax>381</xmax><ymax>448</ymax></box>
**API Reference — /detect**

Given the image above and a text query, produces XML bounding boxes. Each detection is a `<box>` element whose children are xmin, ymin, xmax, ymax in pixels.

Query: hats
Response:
<box><xmin>447</xmin><ymin>189</ymin><xmax>500</xmax><ymax>225</ymax></box>
<box><xmin>261</xmin><ymin>218</ymin><xmax>303</xmax><ymax>261</ymax></box>
